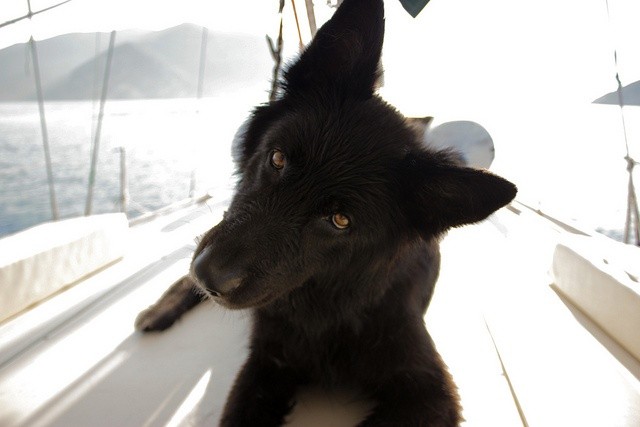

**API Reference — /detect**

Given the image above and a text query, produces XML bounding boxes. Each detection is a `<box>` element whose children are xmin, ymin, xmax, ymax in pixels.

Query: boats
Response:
<box><xmin>0</xmin><ymin>184</ymin><xmax>639</xmax><ymax>427</ymax></box>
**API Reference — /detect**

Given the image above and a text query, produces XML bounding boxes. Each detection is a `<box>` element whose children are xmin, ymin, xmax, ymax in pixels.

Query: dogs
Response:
<box><xmin>133</xmin><ymin>0</ymin><xmax>518</xmax><ymax>426</ymax></box>
